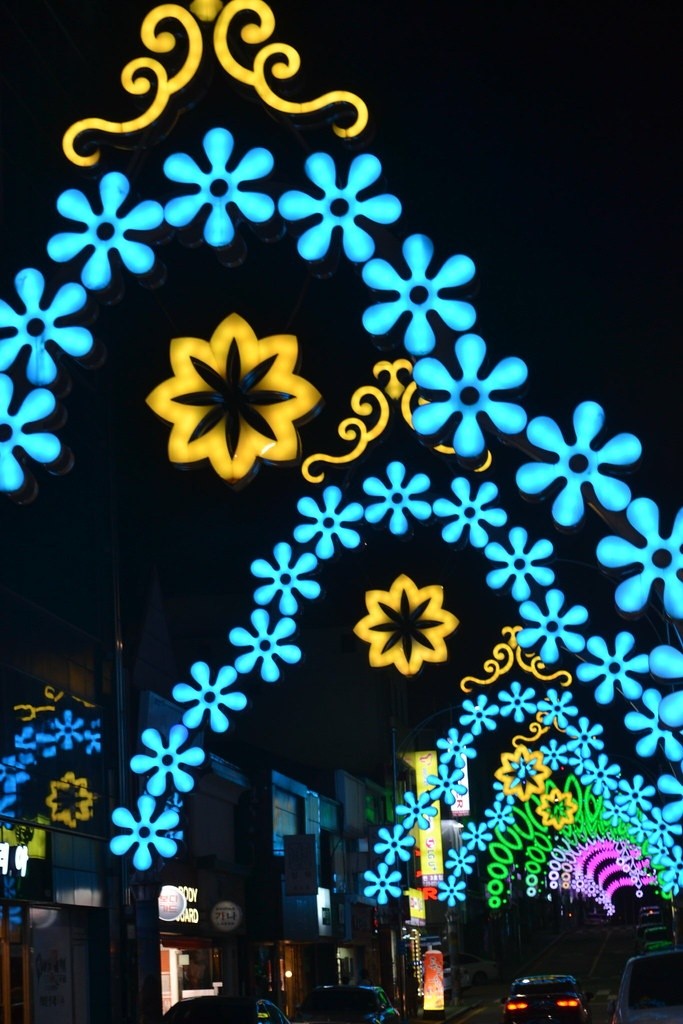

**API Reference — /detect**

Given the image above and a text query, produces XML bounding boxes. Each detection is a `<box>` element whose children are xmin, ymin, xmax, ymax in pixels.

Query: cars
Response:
<box><xmin>156</xmin><ymin>994</ymin><xmax>292</xmax><ymax>1024</ymax></box>
<box><xmin>500</xmin><ymin>973</ymin><xmax>593</xmax><ymax>1024</ymax></box>
<box><xmin>286</xmin><ymin>984</ymin><xmax>401</xmax><ymax>1024</ymax></box>
<box><xmin>633</xmin><ymin>904</ymin><xmax>673</xmax><ymax>954</ymax></box>
<box><xmin>608</xmin><ymin>947</ymin><xmax>683</xmax><ymax>1024</ymax></box>
